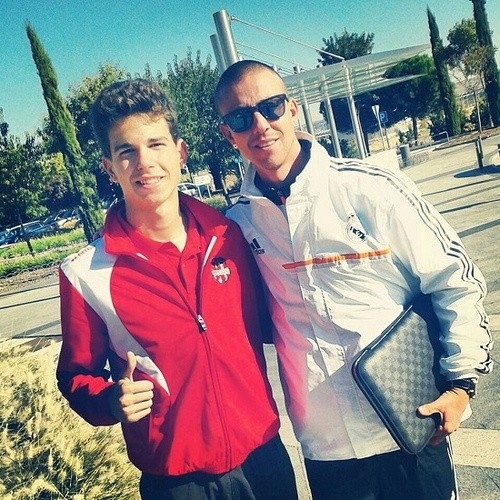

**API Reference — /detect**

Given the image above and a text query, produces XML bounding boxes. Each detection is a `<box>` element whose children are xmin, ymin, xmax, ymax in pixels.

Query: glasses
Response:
<box><xmin>222</xmin><ymin>94</ymin><xmax>290</xmax><ymax>134</ymax></box>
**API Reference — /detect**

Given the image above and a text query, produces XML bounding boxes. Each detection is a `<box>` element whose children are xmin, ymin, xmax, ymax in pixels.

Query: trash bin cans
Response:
<box><xmin>399</xmin><ymin>143</ymin><xmax>412</xmax><ymax>167</ymax></box>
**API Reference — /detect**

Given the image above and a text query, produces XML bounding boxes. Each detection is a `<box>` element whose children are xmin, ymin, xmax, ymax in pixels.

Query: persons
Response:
<box><xmin>56</xmin><ymin>80</ymin><xmax>299</xmax><ymax>500</ymax></box>
<box><xmin>213</xmin><ymin>59</ymin><xmax>493</xmax><ymax>500</ymax></box>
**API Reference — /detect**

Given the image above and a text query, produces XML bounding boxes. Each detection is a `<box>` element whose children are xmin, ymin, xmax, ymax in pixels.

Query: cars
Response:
<box><xmin>178</xmin><ymin>183</ymin><xmax>198</xmax><ymax>196</ymax></box>
<box><xmin>0</xmin><ymin>200</ymin><xmax>109</xmax><ymax>246</ymax></box>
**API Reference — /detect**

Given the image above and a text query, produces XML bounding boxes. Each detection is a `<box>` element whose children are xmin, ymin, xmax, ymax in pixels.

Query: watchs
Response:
<box><xmin>443</xmin><ymin>379</ymin><xmax>478</xmax><ymax>399</ymax></box>
